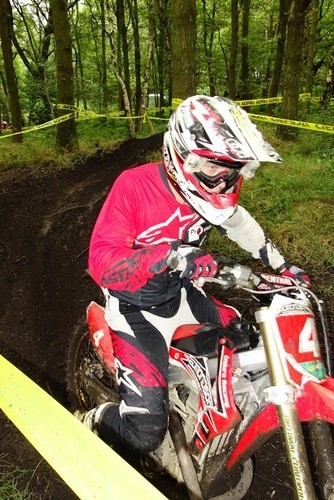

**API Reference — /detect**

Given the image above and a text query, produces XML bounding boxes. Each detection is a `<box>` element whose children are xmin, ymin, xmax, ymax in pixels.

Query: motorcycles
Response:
<box><xmin>61</xmin><ymin>239</ymin><xmax>333</xmax><ymax>500</ymax></box>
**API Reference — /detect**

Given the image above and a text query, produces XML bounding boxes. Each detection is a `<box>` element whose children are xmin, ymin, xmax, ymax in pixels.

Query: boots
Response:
<box><xmin>74</xmin><ymin>402</ymin><xmax>116</xmax><ymax>451</ymax></box>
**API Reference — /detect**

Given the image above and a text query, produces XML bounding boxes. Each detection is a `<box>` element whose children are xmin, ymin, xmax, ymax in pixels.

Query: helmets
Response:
<box><xmin>162</xmin><ymin>94</ymin><xmax>282</xmax><ymax>226</ymax></box>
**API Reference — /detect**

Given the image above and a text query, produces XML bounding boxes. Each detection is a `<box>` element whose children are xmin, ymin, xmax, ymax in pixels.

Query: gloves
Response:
<box><xmin>276</xmin><ymin>261</ymin><xmax>312</xmax><ymax>290</ymax></box>
<box><xmin>164</xmin><ymin>239</ymin><xmax>218</xmax><ymax>281</ymax></box>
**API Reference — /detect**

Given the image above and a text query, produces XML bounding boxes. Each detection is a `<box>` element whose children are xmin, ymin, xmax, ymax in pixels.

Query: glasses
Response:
<box><xmin>191</xmin><ymin>158</ymin><xmax>245</xmax><ymax>189</ymax></box>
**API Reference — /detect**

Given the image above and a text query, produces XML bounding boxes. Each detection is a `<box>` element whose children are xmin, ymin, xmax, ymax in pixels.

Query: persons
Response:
<box><xmin>69</xmin><ymin>93</ymin><xmax>313</xmax><ymax>454</ymax></box>
